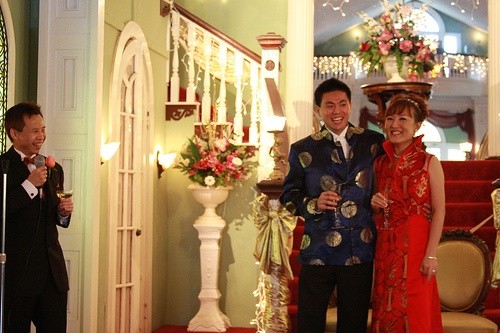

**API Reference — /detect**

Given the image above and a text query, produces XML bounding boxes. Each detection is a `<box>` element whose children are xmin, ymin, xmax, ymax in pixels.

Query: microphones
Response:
<box><xmin>35</xmin><ymin>154</ymin><xmax>46</xmax><ymax>196</ymax></box>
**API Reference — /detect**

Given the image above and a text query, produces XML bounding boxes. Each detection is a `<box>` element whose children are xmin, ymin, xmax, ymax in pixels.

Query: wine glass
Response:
<box><xmin>55</xmin><ymin>177</ymin><xmax>74</xmax><ymax>225</ymax></box>
<box><xmin>326</xmin><ymin>182</ymin><xmax>345</xmax><ymax>229</ymax></box>
<box><xmin>379</xmin><ymin>183</ymin><xmax>394</xmax><ymax>231</ymax></box>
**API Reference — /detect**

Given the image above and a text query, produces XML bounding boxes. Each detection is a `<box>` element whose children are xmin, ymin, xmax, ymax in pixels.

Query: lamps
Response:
<box><xmin>460</xmin><ymin>142</ymin><xmax>474</xmax><ymax>160</ymax></box>
<box><xmin>263</xmin><ymin>117</ymin><xmax>289</xmax><ymax>180</ymax></box>
<box><xmin>157</xmin><ymin>151</ymin><xmax>176</xmax><ymax>181</ymax></box>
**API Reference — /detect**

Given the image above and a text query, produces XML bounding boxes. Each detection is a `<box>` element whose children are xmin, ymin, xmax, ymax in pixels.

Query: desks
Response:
<box><xmin>362</xmin><ymin>81</ymin><xmax>433</xmax><ymax>129</ymax></box>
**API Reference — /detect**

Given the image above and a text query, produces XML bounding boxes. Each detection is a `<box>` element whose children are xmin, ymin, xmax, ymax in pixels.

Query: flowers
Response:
<box><xmin>178</xmin><ymin>137</ymin><xmax>252</xmax><ymax>188</ymax></box>
<box><xmin>359</xmin><ymin>1</ymin><xmax>441</xmax><ymax>79</ymax></box>
<box><xmin>46</xmin><ymin>155</ymin><xmax>57</xmax><ymax>170</ymax></box>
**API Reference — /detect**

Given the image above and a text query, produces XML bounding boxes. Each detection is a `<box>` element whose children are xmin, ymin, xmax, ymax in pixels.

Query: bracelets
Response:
<box><xmin>422</xmin><ymin>256</ymin><xmax>438</xmax><ymax>260</ymax></box>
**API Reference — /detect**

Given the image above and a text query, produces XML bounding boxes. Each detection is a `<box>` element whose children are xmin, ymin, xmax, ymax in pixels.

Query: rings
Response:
<box><xmin>432</xmin><ymin>270</ymin><xmax>436</xmax><ymax>272</ymax></box>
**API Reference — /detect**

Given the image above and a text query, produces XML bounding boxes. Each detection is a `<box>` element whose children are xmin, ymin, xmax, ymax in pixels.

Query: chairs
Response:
<box><xmin>325</xmin><ymin>260</ymin><xmax>373</xmax><ymax>333</ymax></box>
<box><xmin>434</xmin><ymin>225</ymin><xmax>498</xmax><ymax>333</ymax></box>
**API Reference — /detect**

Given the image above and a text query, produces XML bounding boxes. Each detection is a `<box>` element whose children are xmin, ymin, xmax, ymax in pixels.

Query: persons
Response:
<box><xmin>0</xmin><ymin>102</ymin><xmax>75</xmax><ymax>333</ymax></box>
<box><xmin>371</xmin><ymin>92</ymin><xmax>446</xmax><ymax>333</ymax></box>
<box><xmin>279</xmin><ymin>75</ymin><xmax>435</xmax><ymax>333</ymax></box>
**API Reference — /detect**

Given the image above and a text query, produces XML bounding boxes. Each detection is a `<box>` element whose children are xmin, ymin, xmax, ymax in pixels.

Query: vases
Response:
<box><xmin>380</xmin><ymin>55</ymin><xmax>413</xmax><ymax>81</ymax></box>
<box><xmin>188</xmin><ymin>182</ymin><xmax>230</xmax><ymax>223</ymax></box>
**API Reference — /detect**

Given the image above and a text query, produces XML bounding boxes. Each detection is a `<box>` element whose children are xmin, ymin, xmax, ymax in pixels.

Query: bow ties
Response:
<box><xmin>24</xmin><ymin>156</ymin><xmax>35</xmax><ymax>165</ymax></box>
